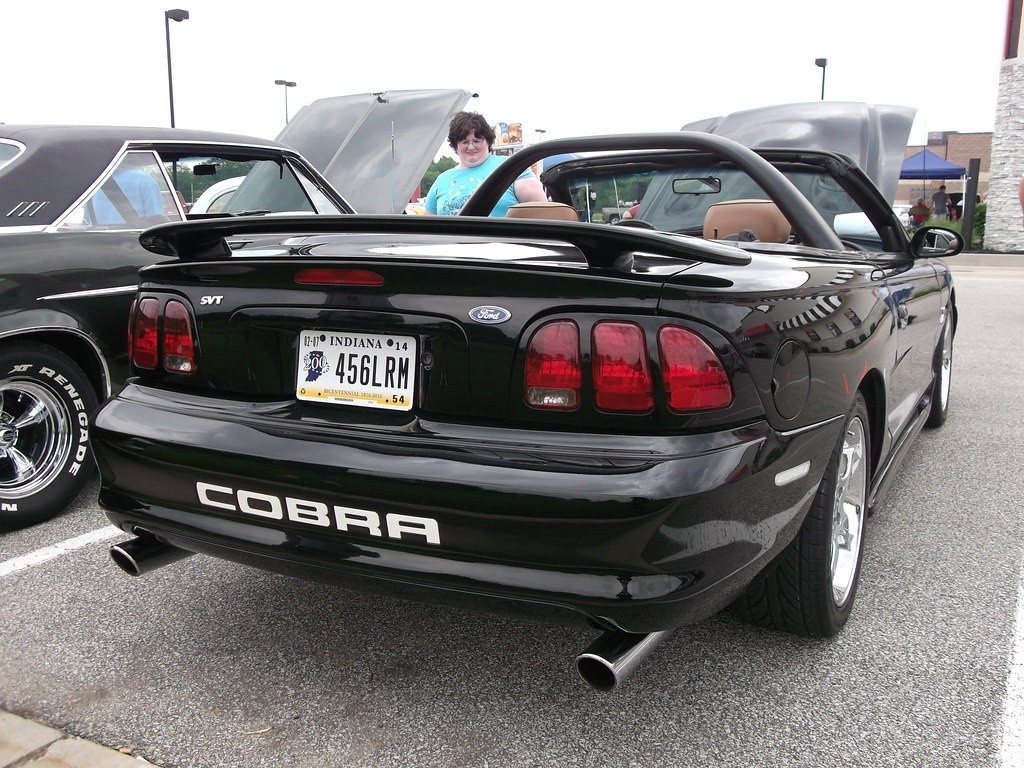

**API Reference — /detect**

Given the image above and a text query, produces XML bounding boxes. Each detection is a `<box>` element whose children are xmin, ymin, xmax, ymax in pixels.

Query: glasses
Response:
<box><xmin>455</xmin><ymin>136</ymin><xmax>486</xmax><ymax>147</ymax></box>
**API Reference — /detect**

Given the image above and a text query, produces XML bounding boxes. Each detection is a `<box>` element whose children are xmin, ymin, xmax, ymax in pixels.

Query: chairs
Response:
<box><xmin>505</xmin><ymin>202</ymin><xmax>582</xmax><ymax>222</ymax></box>
<box><xmin>907</xmin><ymin>207</ymin><xmax>931</xmax><ymax>235</ymax></box>
<box><xmin>947</xmin><ymin>205</ymin><xmax>963</xmax><ymax>222</ymax></box>
<box><xmin>703</xmin><ymin>199</ymin><xmax>792</xmax><ymax>244</ymax></box>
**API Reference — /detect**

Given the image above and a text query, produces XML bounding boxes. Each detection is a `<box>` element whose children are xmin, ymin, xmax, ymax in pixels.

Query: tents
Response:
<box><xmin>543</xmin><ymin>154</ymin><xmax>621</xmax><ymax>223</ymax></box>
<box><xmin>899</xmin><ymin>149</ymin><xmax>968</xmax><ymax>220</ymax></box>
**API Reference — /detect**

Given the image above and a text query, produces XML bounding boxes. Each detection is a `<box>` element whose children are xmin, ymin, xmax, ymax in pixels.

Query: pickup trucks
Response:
<box><xmin>601</xmin><ymin>206</ymin><xmax>631</xmax><ymax>226</ymax></box>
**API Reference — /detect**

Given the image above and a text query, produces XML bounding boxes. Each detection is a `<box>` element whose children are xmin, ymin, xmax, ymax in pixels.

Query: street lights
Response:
<box><xmin>815</xmin><ymin>58</ymin><xmax>828</xmax><ymax>101</ymax></box>
<box><xmin>534</xmin><ymin>128</ymin><xmax>546</xmax><ymax>140</ymax></box>
<box><xmin>274</xmin><ymin>80</ymin><xmax>297</xmax><ymax>125</ymax></box>
<box><xmin>164</xmin><ymin>7</ymin><xmax>189</xmax><ymax>193</ymax></box>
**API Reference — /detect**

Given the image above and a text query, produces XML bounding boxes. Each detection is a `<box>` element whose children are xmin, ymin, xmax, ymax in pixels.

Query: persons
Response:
<box><xmin>931</xmin><ymin>185</ymin><xmax>952</xmax><ymax>222</ymax></box>
<box><xmin>84</xmin><ymin>168</ymin><xmax>163</xmax><ymax>224</ymax></box>
<box><xmin>912</xmin><ymin>197</ymin><xmax>929</xmax><ymax>227</ymax></box>
<box><xmin>424</xmin><ymin>111</ymin><xmax>547</xmax><ymax>217</ymax></box>
<box><xmin>1019</xmin><ymin>173</ymin><xmax>1024</xmax><ymax>226</ymax></box>
<box><xmin>581</xmin><ymin>184</ymin><xmax>597</xmax><ymax>221</ymax></box>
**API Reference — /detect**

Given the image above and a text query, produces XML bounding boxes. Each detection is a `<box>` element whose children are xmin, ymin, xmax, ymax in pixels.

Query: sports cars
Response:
<box><xmin>90</xmin><ymin>100</ymin><xmax>965</xmax><ymax>697</ymax></box>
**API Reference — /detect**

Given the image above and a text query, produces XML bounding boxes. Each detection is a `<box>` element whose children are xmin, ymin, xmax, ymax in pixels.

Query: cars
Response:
<box><xmin>622</xmin><ymin>203</ymin><xmax>642</xmax><ymax>224</ymax></box>
<box><xmin>1</xmin><ymin>86</ymin><xmax>480</xmax><ymax>531</ymax></box>
